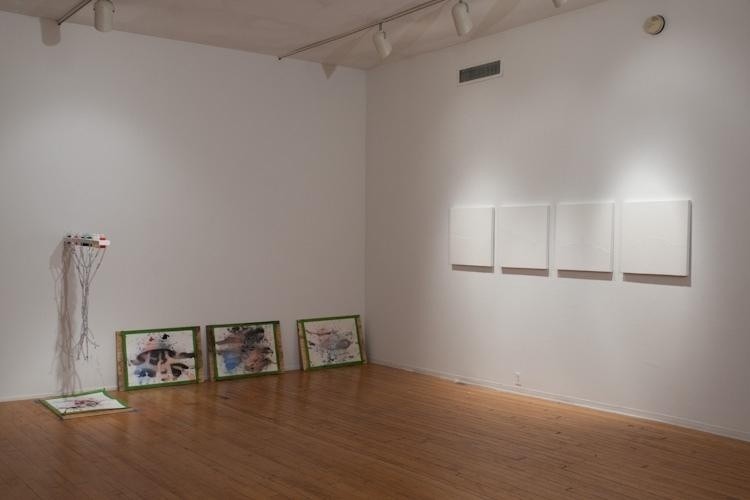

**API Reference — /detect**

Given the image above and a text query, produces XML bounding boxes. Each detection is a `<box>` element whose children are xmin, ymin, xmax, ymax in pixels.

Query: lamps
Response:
<box><xmin>278</xmin><ymin>0</ymin><xmax>568</xmax><ymax>60</ymax></box>
<box><xmin>58</xmin><ymin>0</ymin><xmax>115</xmax><ymax>32</ymax></box>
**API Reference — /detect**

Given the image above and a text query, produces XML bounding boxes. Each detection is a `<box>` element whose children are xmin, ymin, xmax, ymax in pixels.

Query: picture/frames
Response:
<box><xmin>116</xmin><ymin>315</ymin><xmax>369</xmax><ymax>394</ymax></box>
